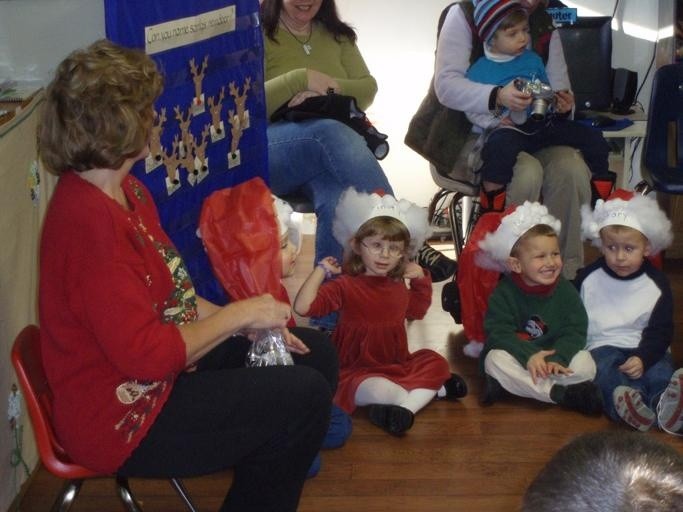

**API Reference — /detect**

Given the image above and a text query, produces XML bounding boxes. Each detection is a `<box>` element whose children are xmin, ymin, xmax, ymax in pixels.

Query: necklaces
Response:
<box><xmin>276</xmin><ymin>13</ymin><xmax>315</xmax><ymax>57</ymax></box>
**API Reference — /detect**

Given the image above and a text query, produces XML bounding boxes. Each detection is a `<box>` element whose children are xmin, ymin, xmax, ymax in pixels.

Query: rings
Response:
<box><xmin>326</xmin><ymin>87</ymin><xmax>334</xmax><ymax>95</ymax></box>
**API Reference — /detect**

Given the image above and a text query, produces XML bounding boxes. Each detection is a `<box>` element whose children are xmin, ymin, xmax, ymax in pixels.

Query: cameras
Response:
<box><xmin>517</xmin><ymin>79</ymin><xmax>556</xmax><ymax>120</ymax></box>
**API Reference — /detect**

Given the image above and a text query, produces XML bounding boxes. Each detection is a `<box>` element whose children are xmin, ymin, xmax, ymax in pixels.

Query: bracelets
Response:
<box><xmin>318</xmin><ymin>262</ymin><xmax>333</xmax><ymax>280</ymax></box>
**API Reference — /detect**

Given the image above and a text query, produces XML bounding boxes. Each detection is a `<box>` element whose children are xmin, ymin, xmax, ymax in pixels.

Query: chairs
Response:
<box><xmin>425</xmin><ymin>159</ymin><xmax>480</xmax><ymax>260</ymax></box>
<box><xmin>10</xmin><ymin>322</ymin><xmax>193</xmax><ymax>511</ymax></box>
<box><xmin>635</xmin><ymin>63</ymin><xmax>683</xmax><ymax>197</ymax></box>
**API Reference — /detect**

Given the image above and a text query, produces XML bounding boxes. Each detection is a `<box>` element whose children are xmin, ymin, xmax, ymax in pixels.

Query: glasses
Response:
<box><xmin>360</xmin><ymin>240</ymin><xmax>405</xmax><ymax>260</ymax></box>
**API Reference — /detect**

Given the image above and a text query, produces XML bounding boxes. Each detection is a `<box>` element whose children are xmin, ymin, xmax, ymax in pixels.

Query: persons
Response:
<box><xmin>192</xmin><ymin>176</ymin><xmax>353</xmax><ymax>481</ymax></box>
<box><xmin>404</xmin><ymin>1</ymin><xmax>589</xmax><ymax>287</ymax></box>
<box><xmin>292</xmin><ymin>216</ymin><xmax>468</xmax><ymax>437</ymax></box>
<box><xmin>463</xmin><ymin>1</ymin><xmax>638</xmax><ymax>226</ymax></box>
<box><xmin>478</xmin><ymin>223</ymin><xmax>606</xmax><ymax>416</ymax></box>
<box><xmin>34</xmin><ymin>41</ymin><xmax>337</xmax><ymax>512</ymax></box>
<box><xmin>254</xmin><ymin>1</ymin><xmax>457</xmax><ymax>336</ymax></box>
<box><xmin>572</xmin><ymin>209</ymin><xmax>683</xmax><ymax>436</ymax></box>
<box><xmin>528</xmin><ymin>429</ymin><xmax>683</xmax><ymax>512</ymax></box>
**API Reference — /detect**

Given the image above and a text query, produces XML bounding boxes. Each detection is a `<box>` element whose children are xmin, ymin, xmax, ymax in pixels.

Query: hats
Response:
<box><xmin>471</xmin><ymin>0</ymin><xmax>523</xmax><ymax>45</ymax></box>
<box><xmin>579</xmin><ymin>183</ymin><xmax>673</xmax><ymax>280</ymax></box>
<box><xmin>329</xmin><ymin>185</ymin><xmax>428</xmax><ymax>253</ymax></box>
<box><xmin>193</xmin><ymin>176</ymin><xmax>299</xmax><ymax>371</ymax></box>
<box><xmin>453</xmin><ymin>196</ymin><xmax>563</xmax><ymax>361</ymax></box>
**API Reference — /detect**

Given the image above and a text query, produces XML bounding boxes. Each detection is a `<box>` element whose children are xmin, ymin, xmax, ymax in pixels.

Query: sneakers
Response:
<box><xmin>436</xmin><ymin>373</ymin><xmax>467</xmax><ymax>401</ymax></box>
<box><xmin>369</xmin><ymin>402</ymin><xmax>414</xmax><ymax>435</ymax></box>
<box><xmin>591</xmin><ymin>170</ymin><xmax>617</xmax><ymax>200</ymax></box>
<box><xmin>612</xmin><ymin>385</ymin><xmax>655</xmax><ymax>433</ymax></box>
<box><xmin>656</xmin><ymin>368</ymin><xmax>683</xmax><ymax>435</ymax></box>
<box><xmin>478</xmin><ymin>185</ymin><xmax>507</xmax><ymax>219</ymax></box>
<box><xmin>477</xmin><ymin>369</ymin><xmax>500</xmax><ymax>405</ymax></box>
<box><xmin>563</xmin><ymin>380</ymin><xmax>604</xmax><ymax>418</ymax></box>
<box><xmin>414</xmin><ymin>240</ymin><xmax>456</xmax><ymax>283</ymax></box>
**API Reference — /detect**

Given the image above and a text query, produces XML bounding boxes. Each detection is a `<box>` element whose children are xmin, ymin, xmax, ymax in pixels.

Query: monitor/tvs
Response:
<box><xmin>557</xmin><ymin>15</ymin><xmax>612</xmax><ymax>109</ymax></box>
